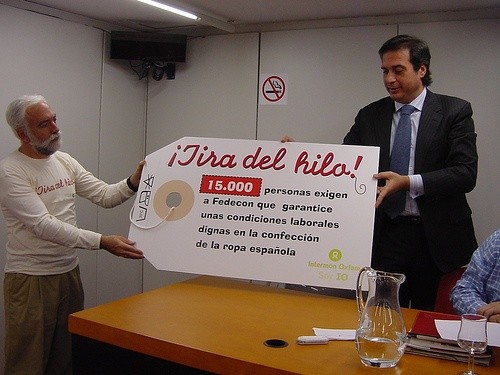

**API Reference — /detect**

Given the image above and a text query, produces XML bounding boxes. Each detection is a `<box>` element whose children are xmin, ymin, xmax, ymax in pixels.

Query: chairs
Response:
<box><xmin>435</xmin><ymin>267</ymin><xmax>468</xmax><ymax>316</ymax></box>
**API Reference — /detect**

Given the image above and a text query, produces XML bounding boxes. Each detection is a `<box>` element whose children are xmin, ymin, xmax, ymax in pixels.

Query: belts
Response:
<box><xmin>392</xmin><ymin>215</ymin><xmax>421</xmax><ymax>221</ymax></box>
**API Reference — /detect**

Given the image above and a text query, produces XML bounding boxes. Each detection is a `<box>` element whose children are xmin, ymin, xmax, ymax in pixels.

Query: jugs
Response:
<box><xmin>355</xmin><ymin>267</ymin><xmax>406</xmax><ymax>369</ymax></box>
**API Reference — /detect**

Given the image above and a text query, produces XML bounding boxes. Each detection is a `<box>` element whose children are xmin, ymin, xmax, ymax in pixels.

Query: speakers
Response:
<box><xmin>110</xmin><ymin>31</ymin><xmax>186</xmax><ymax>63</ymax></box>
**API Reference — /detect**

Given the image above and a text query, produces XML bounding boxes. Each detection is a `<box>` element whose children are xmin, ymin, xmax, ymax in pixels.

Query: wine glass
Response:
<box><xmin>457</xmin><ymin>314</ymin><xmax>487</xmax><ymax>375</ymax></box>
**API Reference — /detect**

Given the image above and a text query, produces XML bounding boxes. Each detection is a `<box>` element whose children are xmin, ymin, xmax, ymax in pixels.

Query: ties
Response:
<box><xmin>382</xmin><ymin>104</ymin><xmax>420</xmax><ymax>222</ymax></box>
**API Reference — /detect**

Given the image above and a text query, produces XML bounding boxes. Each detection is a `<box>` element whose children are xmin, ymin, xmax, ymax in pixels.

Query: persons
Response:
<box><xmin>0</xmin><ymin>95</ymin><xmax>146</xmax><ymax>375</ymax></box>
<box><xmin>281</xmin><ymin>34</ymin><xmax>480</xmax><ymax>316</ymax></box>
<box><xmin>448</xmin><ymin>228</ymin><xmax>500</xmax><ymax>325</ymax></box>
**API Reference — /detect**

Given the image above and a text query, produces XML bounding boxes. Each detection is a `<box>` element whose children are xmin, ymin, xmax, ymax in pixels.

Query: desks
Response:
<box><xmin>69</xmin><ymin>275</ymin><xmax>500</xmax><ymax>375</ymax></box>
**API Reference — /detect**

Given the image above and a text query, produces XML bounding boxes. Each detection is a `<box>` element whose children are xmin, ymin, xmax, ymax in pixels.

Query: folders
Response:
<box><xmin>408</xmin><ymin>310</ymin><xmax>495</xmax><ymax>356</ymax></box>
